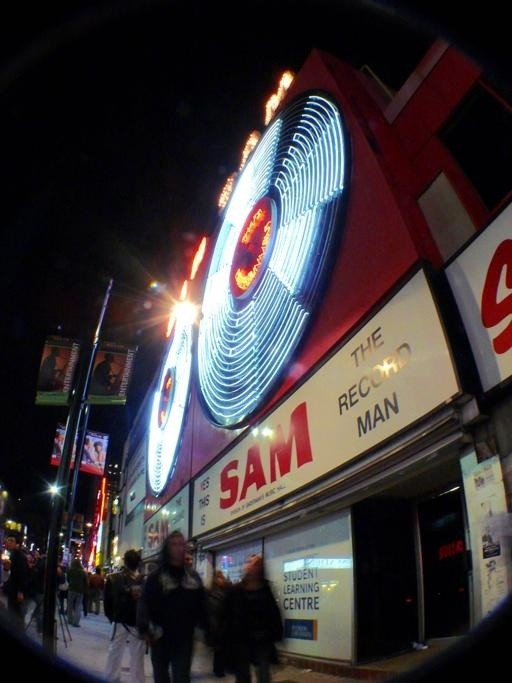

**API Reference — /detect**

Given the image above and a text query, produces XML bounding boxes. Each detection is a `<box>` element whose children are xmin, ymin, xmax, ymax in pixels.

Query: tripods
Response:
<box><xmin>24</xmin><ymin>590</ymin><xmax>73</xmax><ymax>648</ymax></box>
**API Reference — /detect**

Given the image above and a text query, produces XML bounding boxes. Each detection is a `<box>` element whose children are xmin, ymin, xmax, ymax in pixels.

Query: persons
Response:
<box><xmin>0</xmin><ymin>531</ymin><xmax>104</xmax><ymax>635</ymax></box>
<box><xmin>102</xmin><ymin>530</ymin><xmax>287</xmax><ymax>683</ymax></box>
